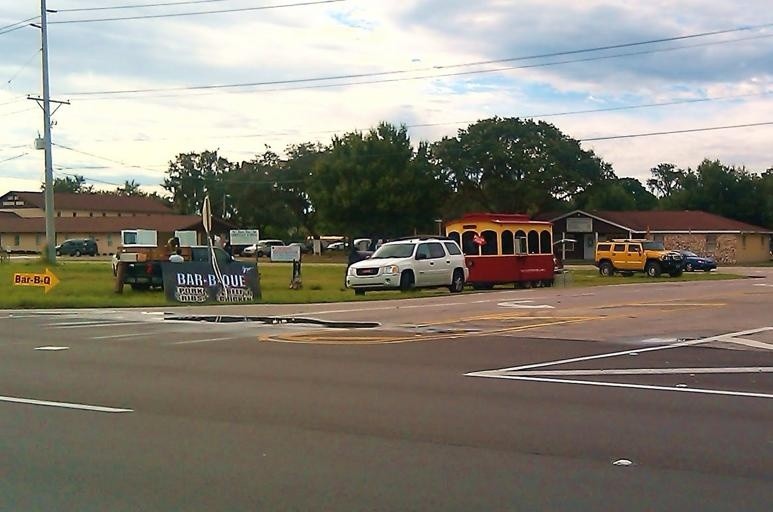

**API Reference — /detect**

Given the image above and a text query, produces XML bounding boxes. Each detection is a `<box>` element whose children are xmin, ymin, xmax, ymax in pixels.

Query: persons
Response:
<box><xmin>224</xmin><ymin>240</ymin><xmax>233</xmax><ymax>256</ymax></box>
<box><xmin>169</xmin><ymin>248</ymin><xmax>184</xmax><ymax>263</ymax></box>
<box><xmin>167</xmin><ymin>237</ymin><xmax>180</xmax><ymax>248</ymax></box>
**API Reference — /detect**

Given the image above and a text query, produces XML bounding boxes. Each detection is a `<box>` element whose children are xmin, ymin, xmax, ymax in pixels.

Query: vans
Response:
<box><xmin>55</xmin><ymin>239</ymin><xmax>97</xmax><ymax>257</ymax></box>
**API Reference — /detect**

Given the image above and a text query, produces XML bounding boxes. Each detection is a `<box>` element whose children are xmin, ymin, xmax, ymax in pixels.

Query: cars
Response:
<box><xmin>326</xmin><ymin>238</ymin><xmax>386</xmax><ymax>252</ymax></box>
<box><xmin>288</xmin><ymin>243</ymin><xmax>311</xmax><ymax>253</ymax></box>
<box><xmin>109</xmin><ymin>252</ymin><xmax>120</xmax><ymax>277</ymax></box>
<box><xmin>673</xmin><ymin>248</ymin><xmax>716</xmax><ymax>273</ymax></box>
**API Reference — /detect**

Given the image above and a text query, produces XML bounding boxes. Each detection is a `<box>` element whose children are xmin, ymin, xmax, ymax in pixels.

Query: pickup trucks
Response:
<box><xmin>122</xmin><ymin>245</ymin><xmax>240</xmax><ymax>290</ymax></box>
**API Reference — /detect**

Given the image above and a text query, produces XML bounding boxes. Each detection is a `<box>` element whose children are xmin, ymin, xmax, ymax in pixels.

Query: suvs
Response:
<box><xmin>593</xmin><ymin>237</ymin><xmax>683</xmax><ymax>278</ymax></box>
<box><xmin>244</xmin><ymin>239</ymin><xmax>285</xmax><ymax>257</ymax></box>
<box><xmin>345</xmin><ymin>236</ymin><xmax>469</xmax><ymax>296</ymax></box>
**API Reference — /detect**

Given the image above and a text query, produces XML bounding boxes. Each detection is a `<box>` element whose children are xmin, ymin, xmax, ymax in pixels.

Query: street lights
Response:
<box><xmin>435</xmin><ymin>220</ymin><xmax>442</xmax><ymax>235</ymax></box>
